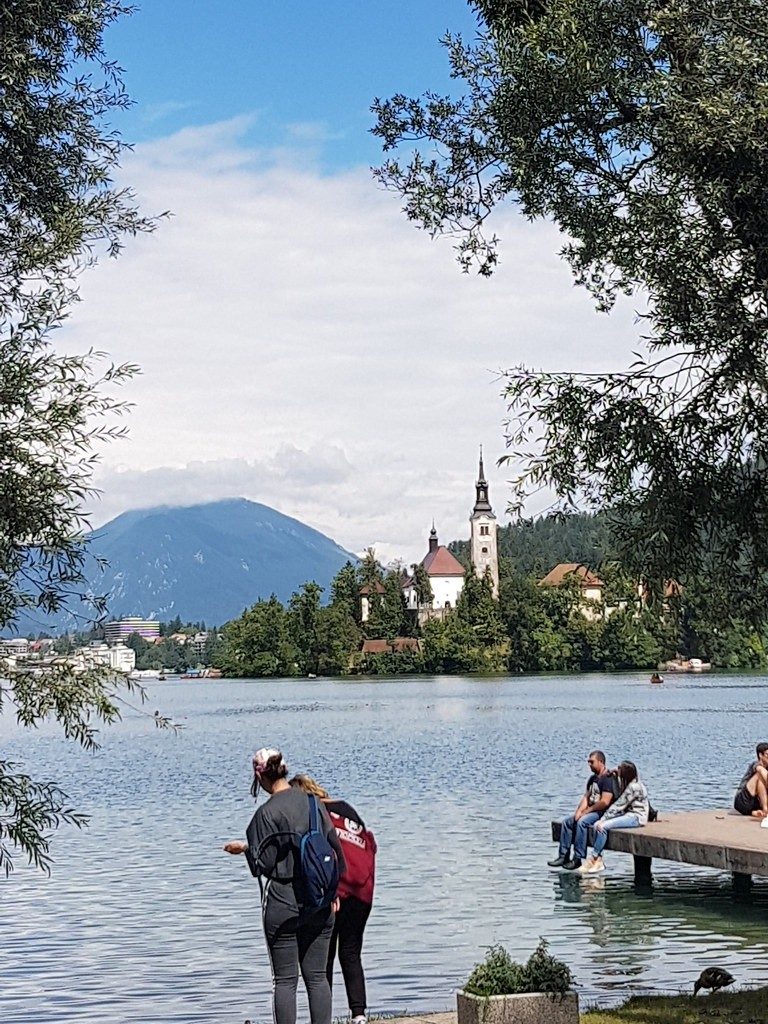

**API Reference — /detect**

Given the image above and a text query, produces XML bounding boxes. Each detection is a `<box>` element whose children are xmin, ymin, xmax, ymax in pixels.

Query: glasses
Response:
<box><xmin>587</xmin><ymin>760</ymin><xmax>594</xmax><ymax>764</ymax></box>
<box><xmin>618</xmin><ymin>765</ymin><xmax>622</xmax><ymax>770</ymax></box>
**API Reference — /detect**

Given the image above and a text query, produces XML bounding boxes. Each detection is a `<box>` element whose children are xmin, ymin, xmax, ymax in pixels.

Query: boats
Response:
<box><xmin>650</xmin><ymin>673</ymin><xmax>664</xmax><ymax>683</ymax></box>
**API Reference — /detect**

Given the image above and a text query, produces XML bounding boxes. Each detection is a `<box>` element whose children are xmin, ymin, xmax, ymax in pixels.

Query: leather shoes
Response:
<box><xmin>547</xmin><ymin>857</ymin><xmax>571</xmax><ymax>867</ymax></box>
<box><xmin>563</xmin><ymin>857</ymin><xmax>582</xmax><ymax>869</ymax></box>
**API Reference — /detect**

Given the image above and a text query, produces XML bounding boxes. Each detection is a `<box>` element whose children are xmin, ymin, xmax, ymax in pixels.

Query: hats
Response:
<box><xmin>252</xmin><ymin>745</ymin><xmax>284</xmax><ymax>774</ymax></box>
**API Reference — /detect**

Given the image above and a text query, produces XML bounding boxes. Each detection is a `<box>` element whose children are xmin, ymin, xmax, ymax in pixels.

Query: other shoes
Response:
<box><xmin>353</xmin><ymin>1018</ymin><xmax>369</xmax><ymax>1024</ymax></box>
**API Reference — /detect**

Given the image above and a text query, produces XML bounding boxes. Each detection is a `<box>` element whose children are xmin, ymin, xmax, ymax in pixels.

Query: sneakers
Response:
<box><xmin>582</xmin><ymin>880</ymin><xmax>605</xmax><ymax>895</ymax></box>
<box><xmin>589</xmin><ymin>862</ymin><xmax>605</xmax><ymax>872</ymax></box>
<box><xmin>576</xmin><ymin>862</ymin><xmax>594</xmax><ymax>873</ymax></box>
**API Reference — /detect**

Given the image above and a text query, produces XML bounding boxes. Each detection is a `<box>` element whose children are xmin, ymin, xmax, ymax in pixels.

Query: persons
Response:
<box><xmin>225</xmin><ymin>745</ymin><xmax>378</xmax><ymax>1024</ymax></box>
<box><xmin>547</xmin><ymin>751</ymin><xmax>650</xmax><ymax>875</ymax></box>
<box><xmin>733</xmin><ymin>742</ymin><xmax>768</xmax><ymax>816</ymax></box>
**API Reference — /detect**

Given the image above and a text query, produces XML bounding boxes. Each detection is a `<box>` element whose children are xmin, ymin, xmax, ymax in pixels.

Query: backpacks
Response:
<box><xmin>256</xmin><ymin>794</ymin><xmax>340</xmax><ymax>915</ymax></box>
